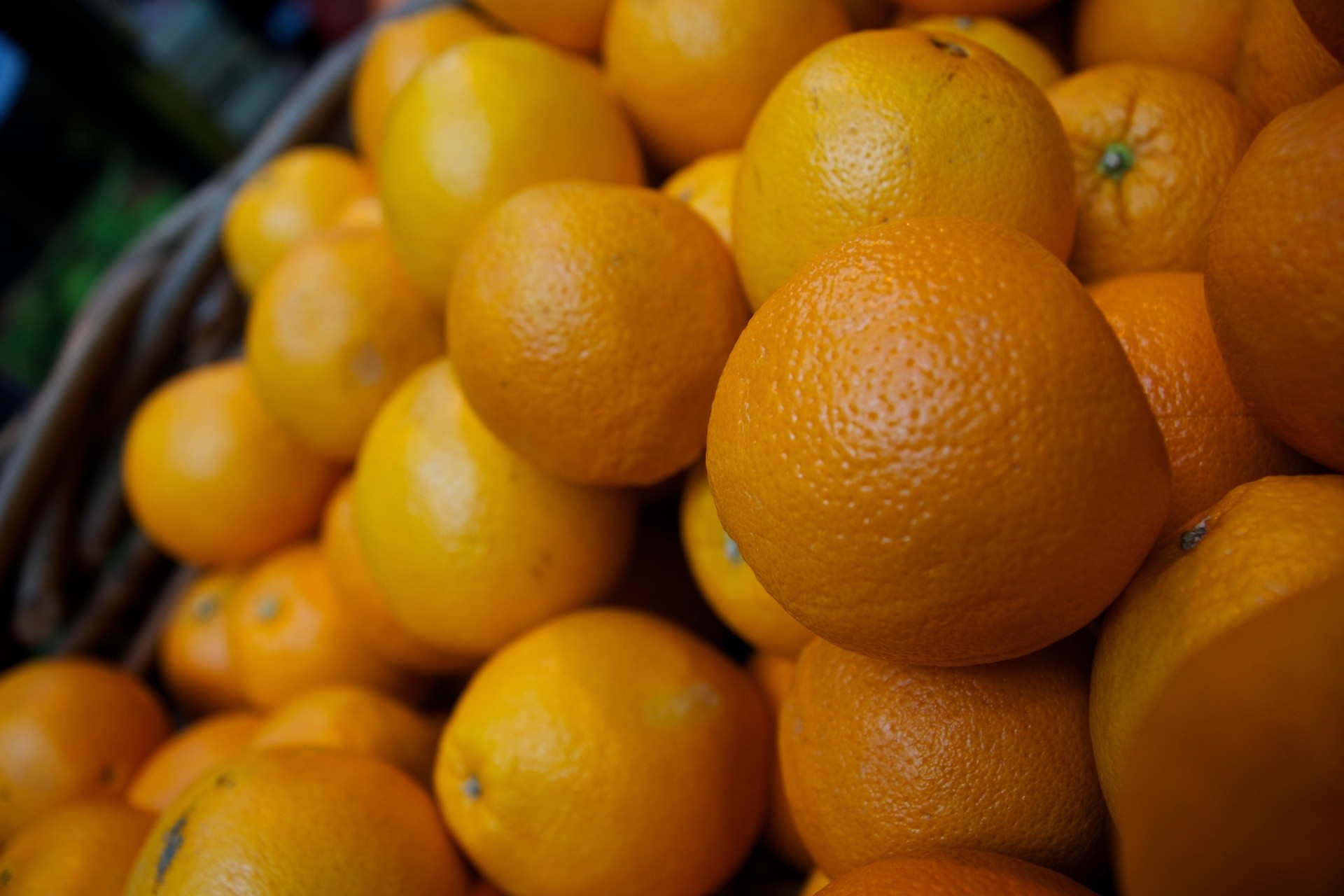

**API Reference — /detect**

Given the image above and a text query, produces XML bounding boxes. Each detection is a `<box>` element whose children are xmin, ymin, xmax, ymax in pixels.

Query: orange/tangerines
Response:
<box><xmin>0</xmin><ymin>0</ymin><xmax>1344</xmax><ymax>896</ymax></box>
